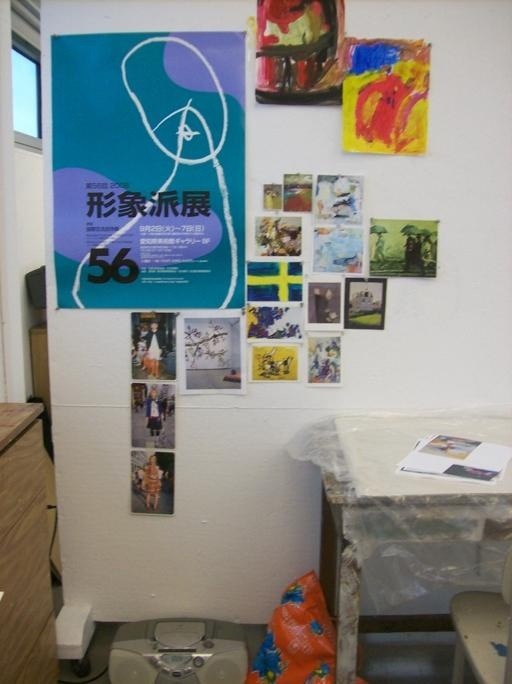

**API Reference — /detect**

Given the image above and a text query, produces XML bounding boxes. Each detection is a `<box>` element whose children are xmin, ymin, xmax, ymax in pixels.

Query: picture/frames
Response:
<box><xmin>342</xmin><ymin>275</ymin><xmax>390</xmax><ymax>330</ymax></box>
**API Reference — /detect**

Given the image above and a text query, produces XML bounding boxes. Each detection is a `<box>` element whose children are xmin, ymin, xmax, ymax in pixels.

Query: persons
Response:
<box><xmin>402</xmin><ymin>231</ymin><xmax>437</xmax><ymax>275</ymax></box>
<box><xmin>132</xmin><ymin>392</ymin><xmax>175</xmax><ymax>420</ymax></box>
<box><xmin>133</xmin><ymin>319</ymin><xmax>165</xmax><ymax>380</ymax></box>
<box><xmin>225</xmin><ymin>369</ymin><xmax>240</xmax><ymax>382</ymax></box>
<box><xmin>373</xmin><ymin>232</ymin><xmax>385</xmax><ymax>263</ymax></box>
<box><xmin>317</xmin><ymin>288</ymin><xmax>334</xmax><ymax>323</ymax></box>
<box><xmin>140</xmin><ymin>454</ymin><xmax>162</xmax><ymax>513</ymax></box>
<box><xmin>133</xmin><ymin>468</ymin><xmax>172</xmax><ymax>496</ymax></box>
<box><xmin>309</xmin><ymin>338</ymin><xmax>340</xmax><ymax>383</ymax></box>
<box><xmin>313</xmin><ymin>286</ymin><xmax>321</xmax><ymax>317</ymax></box>
<box><xmin>145</xmin><ymin>388</ymin><xmax>164</xmax><ymax>444</ymax></box>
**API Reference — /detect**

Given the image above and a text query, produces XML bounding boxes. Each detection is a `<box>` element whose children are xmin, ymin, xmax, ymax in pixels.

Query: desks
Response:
<box><xmin>317</xmin><ymin>410</ymin><xmax>510</xmax><ymax>682</ymax></box>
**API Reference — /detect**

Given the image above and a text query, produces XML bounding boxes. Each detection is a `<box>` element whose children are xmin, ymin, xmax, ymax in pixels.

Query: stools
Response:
<box><xmin>447</xmin><ymin>584</ymin><xmax>510</xmax><ymax>683</ymax></box>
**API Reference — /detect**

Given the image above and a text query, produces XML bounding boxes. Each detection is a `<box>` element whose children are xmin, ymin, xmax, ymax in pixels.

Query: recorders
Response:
<box><xmin>108</xmin><ymin>617</ymin><xmax>248</xmax><ymax>684</ymax></box>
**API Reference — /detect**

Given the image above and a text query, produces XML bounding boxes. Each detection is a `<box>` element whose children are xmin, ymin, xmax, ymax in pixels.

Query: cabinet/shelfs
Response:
<box><xmin>0</xmin><ymin>401</ymin><xmax>65</xmax><ymax>683</ymax></box>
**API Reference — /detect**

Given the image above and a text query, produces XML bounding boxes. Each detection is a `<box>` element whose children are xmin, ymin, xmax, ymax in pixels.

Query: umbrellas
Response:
<box><xmin>371</xmin><ymin>224</ymin><xmax>388</xmax><ymax>233</ymax></box>
<box><xmin>401</xmin><ymin>223</ymin><xmax>431</xmax><ymax>236</ymax></box>
